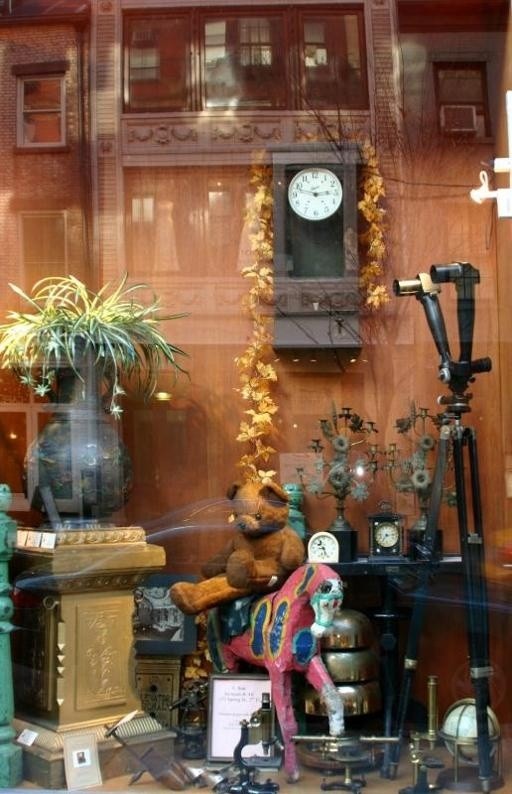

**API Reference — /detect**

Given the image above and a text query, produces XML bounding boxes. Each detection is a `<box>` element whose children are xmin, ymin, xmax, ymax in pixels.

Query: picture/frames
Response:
<box><xmin>62</xmin><ymin>732</ymin><xmax>103</xmax><ymax>791</ymax></box>
<box><xmin>125</xmin><ymin>570</ymin><xmax>199</xmax><ymax>653</ymax></box>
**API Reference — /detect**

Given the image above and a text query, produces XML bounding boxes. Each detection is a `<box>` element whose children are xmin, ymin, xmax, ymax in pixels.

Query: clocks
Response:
<box><xmin>262</xmin><ymin>140</ymin><xmax>368</xmax><ymax>351</ymax></box>
<box><xmin>307</xmin><ymin>531</ymin><xmax>340</xmax><ymax>565</ymax></box>
<box><xmin>367</xmin><ymin>511</ymin><xmax>403</xmax><ymax>557</ymax></box>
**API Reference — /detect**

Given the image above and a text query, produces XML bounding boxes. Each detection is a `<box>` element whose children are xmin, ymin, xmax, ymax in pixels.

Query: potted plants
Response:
<box><xmin>0</xmin><ymin>265</ymin><xmax>193</xmax><ymax>529</ymax></box>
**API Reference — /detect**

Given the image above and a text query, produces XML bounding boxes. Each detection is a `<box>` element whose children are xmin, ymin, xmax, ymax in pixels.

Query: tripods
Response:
<box><xmin>386</xmin><ymin>391</ymin><xmax>492</xmax><ymax>794</ymax></box>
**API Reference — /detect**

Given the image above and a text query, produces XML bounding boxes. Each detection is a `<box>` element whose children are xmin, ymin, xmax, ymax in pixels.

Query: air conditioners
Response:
<box><xmin>440</xmin><ymin>105</ymin><xmax>477</xmax><ymax>135</ymax></box>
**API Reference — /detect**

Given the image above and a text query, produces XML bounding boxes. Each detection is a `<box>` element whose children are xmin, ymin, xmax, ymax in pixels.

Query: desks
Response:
<box><xmin>253</xmin><ymin>551</ymin><xmax>464</xmax><ymax>779</ymax></box>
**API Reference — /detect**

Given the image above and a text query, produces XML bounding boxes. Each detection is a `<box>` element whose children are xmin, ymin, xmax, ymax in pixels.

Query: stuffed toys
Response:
<box><xmin>166</xmin><ymin>479</ymin><xmax>308</xmax><ymax>617</ymax></box>
